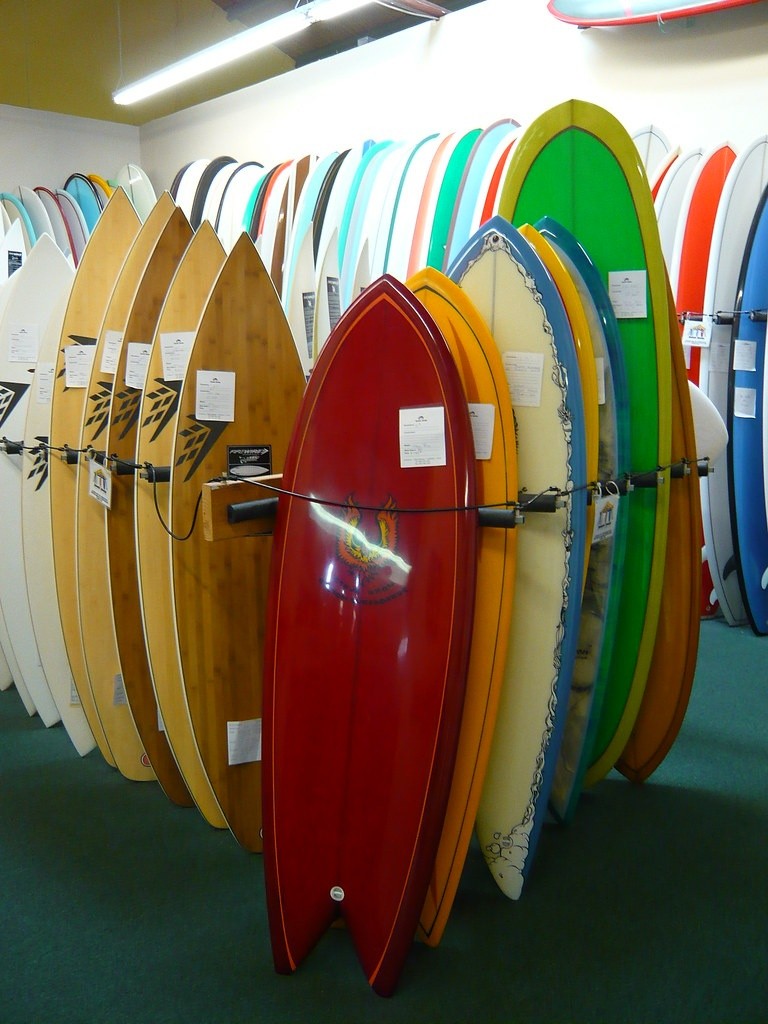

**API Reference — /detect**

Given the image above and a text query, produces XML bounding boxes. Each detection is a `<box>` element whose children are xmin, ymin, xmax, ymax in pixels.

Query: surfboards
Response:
<box><xmin>1</xmin><ymin>98</ymin><xmax>768</xmax><ymax>1002</ymax></box>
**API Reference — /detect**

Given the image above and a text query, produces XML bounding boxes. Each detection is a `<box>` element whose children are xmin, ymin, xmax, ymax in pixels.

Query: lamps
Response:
<box><xmin>106</xmin><ymin>0</ymin><xmax>379</xmax><ymax>106</ymax></box>
